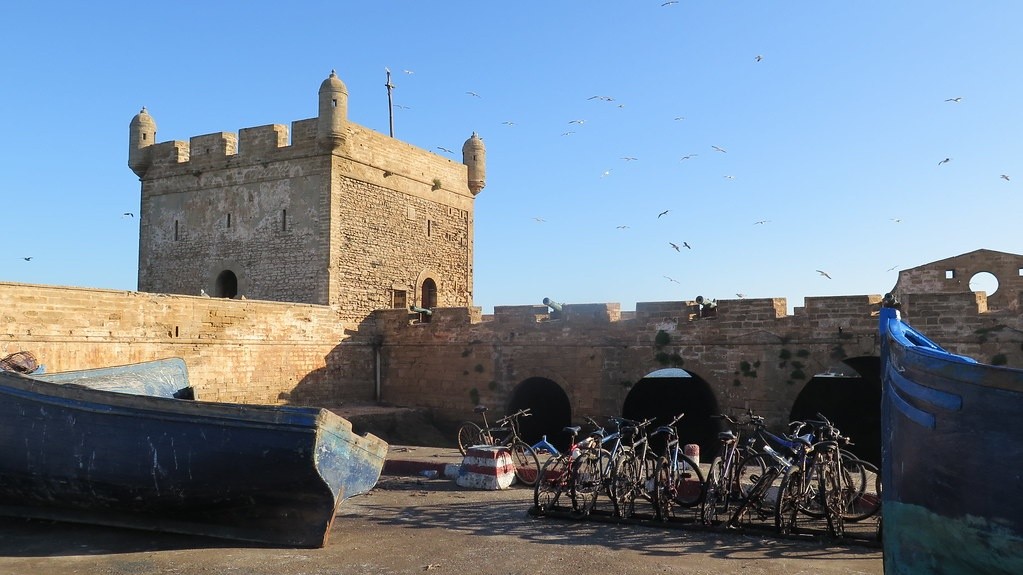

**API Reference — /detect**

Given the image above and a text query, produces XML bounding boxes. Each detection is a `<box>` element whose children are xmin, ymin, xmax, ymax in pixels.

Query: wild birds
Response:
<box><xmin>395</xmin><ymin>0</ymin><xmax>1023</xmax><ymax>284</ymax></box>
<box><xmin>736</xmin><ymin>294</ymin><xmax>746</xmax><ymax>298</ymax></box>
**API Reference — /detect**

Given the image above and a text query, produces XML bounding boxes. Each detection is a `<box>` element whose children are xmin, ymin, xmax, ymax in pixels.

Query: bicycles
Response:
<box><xmin>699</xmin><ymin>407</ymin><xmax>884</xmax><ymax>541</ymax></box>
<box><xmin>456</xmin><ymin>405</ymin><xmax>542</xmax><ymax>487</ymax></box>
<box><xmin>534</xmin><ymin>414</ymin><xmax>707</xmax><ymax>523</ymax></box>
